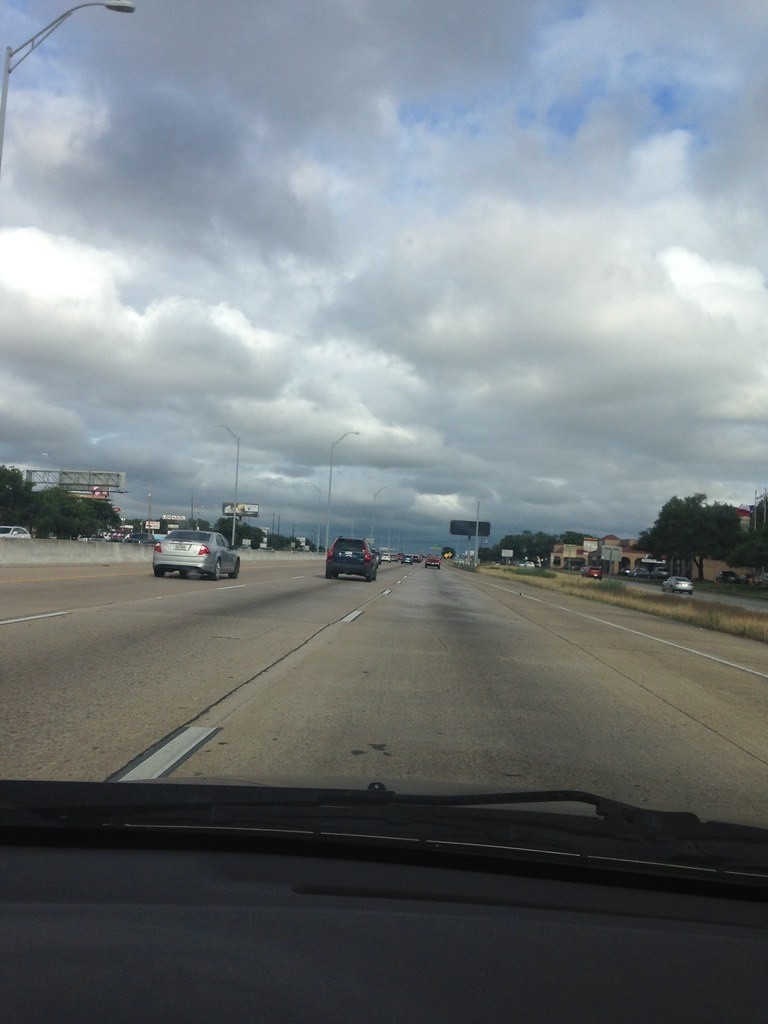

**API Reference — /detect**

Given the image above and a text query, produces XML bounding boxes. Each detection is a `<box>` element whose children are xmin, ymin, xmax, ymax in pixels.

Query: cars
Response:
<box><xmin>390</xmin><ymin>554</ymin><xmax>398</xmax><ymax>562</ymax></box>
<box><xmin>517</xmin><ymin>561</ymin><xmax>526</xmax><ymax>568</ymax></box>
<box><xmin>380</xmin><ymin>552</ymin><xmax>392</xmax><ymax>563</ymax></box>
<box><xmin>619</xmin><ymin>566</ymin><xmax>651</xmax><ymax>579</ymax></box>
<box><xmin>398</xmin><ymin>552</ymin><xmax>423</xmax><ymax>562</ymax></box>
<box><xmin>525</xmin><ymin>561</ymin><xmax>536</xmax><ymax>569</ymax></box>
<box><xmin>662</xmin><ymin>575</ymin><xmax>695</xmax><ymax>595</ymax></box>
<box><xmin>401</xmin><ymin>555</ymin><xmax>413</xmax><ymax>565</ymax></box>
<box><xmin>152</xmin><ymin>529</ymin><xmax>240</xmax><ymax>581</ymax></box>
<box><xmin>582</xmin><ymin>566</ymin><xmax>603</xmax><ymax>580</ymax></box>
<box><xmin>0</xmin><ymin>526</ymin><xmax>31</xmax><ymax>539</ymax></box>
<box><xmin>125</xmin><ymin>533</ymin><xmax>159</xmax><ymax>544</ymax></box>
<box><xmin>424</xmin><ymin>554</ymin><xmax>442</xmax><ymax>569</ymax></box>
<box><xmin>715</xmin><ymin>570</ymin><xmax>768</xmax><ymax>586</ymax></box>
<box><xmin>650</xmin><ymin>566</ymin><xmax>669</xmax><ymax>579</ymax></box>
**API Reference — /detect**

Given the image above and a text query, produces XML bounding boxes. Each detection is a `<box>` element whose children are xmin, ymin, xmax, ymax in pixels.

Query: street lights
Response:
<box><xmin>0</xmin><ymin>1</ymin><xmax>135</xmax><ymax>171</ymax></box>
<box><xmin>322</xmin><ymin>431</ymin><xmax>361</xmax><ymax>553</ymax></box>
<box><xmin>212</xmin><ymin>423</ymin><xmax>240</xmax><ymax>546</ymax></box>
<box><xmin>304</xmin><ymin>480</ymin><xmax>322</xmax><ymax>555</ymax></box>
<box><xmin>371</xmin><ymin>486</ymin><xmax>389</xmax><ymax>549</ymax></box>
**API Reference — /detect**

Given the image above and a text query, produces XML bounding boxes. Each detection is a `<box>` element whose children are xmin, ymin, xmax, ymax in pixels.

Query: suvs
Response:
<box><xmin>325</xmin><ymin>535</ymin><xmax>380</xmax><ymax>583</ymax></box>
